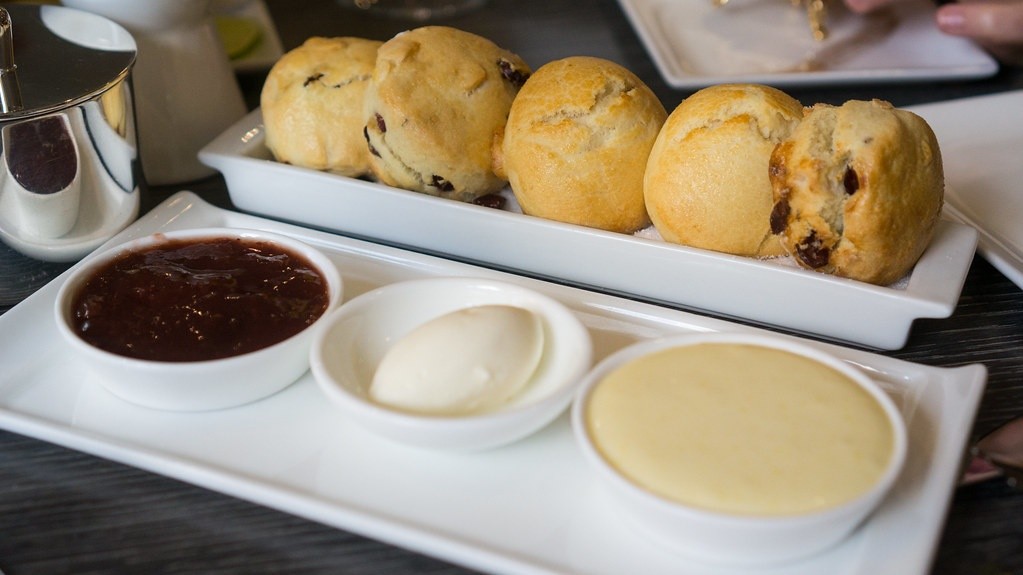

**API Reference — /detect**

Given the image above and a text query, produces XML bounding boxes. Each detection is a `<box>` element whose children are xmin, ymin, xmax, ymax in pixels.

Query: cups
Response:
<box><xmin>54</xmin><ymin>1</ymin><xmax>247</xmax><ymax>185</ymax></box>
<box><xmin>355</xmin><ymin>1</ymin><xmax>487</xmax><ymax>21</ymax></box>
<box><xmin>0</xmin><ymin>0</ymin><xmax>145</xmax><ymax>264</ymax></box>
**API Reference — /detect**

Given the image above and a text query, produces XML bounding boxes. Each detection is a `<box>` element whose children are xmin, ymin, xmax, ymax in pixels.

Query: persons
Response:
<box><xmin>846</xmin><ymin>0</ymin><xmax>1022</xmax><ymax>68</ymax></box>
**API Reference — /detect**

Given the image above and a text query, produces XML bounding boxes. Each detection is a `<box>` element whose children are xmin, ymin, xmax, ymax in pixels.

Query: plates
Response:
<box><xmin>1</xmin><ymin>189</ymin><xmax>987</xmax><ymax>573</ymax></box>
<box><xmin>896</xmin><ymin>88</ymin><xmax>1023</xmax><ymax>290</ymax></box>
<box><xmin>197</xmin><ymin>107</ymin><xmax>982</xmax><ymax>353</ymax></box>
<box><xmin>204</xmin><ymin>0</ymin><xmax>285</xmax><ymax>71</ymax></box>
<box><xmin>617</xmin><ymin>1</ymin><xmax>1001</xmax><ymax>93</ymax></box>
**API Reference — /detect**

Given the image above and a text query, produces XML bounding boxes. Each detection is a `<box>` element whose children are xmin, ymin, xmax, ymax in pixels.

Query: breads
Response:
<box><xmin>258</xmin><ymin>27</ymin><xmax>947</xmax><ymax>288</ymax></box>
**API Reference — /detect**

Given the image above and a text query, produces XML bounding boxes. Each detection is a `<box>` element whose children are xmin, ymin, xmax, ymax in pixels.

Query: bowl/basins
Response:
<box><xmin>570</xmin><ymin>332</ymin><xmax>910</xmax><ymax>558</ymax></box>
<box><xmin>307</xmin><ymin>277</ymin><xmax>593</xmax><ymax>451</ymax></box>
<box><xmin>54</xmin><ymin>226</ymin><xmax>341</xmax><ymax>411</ymax></box>
